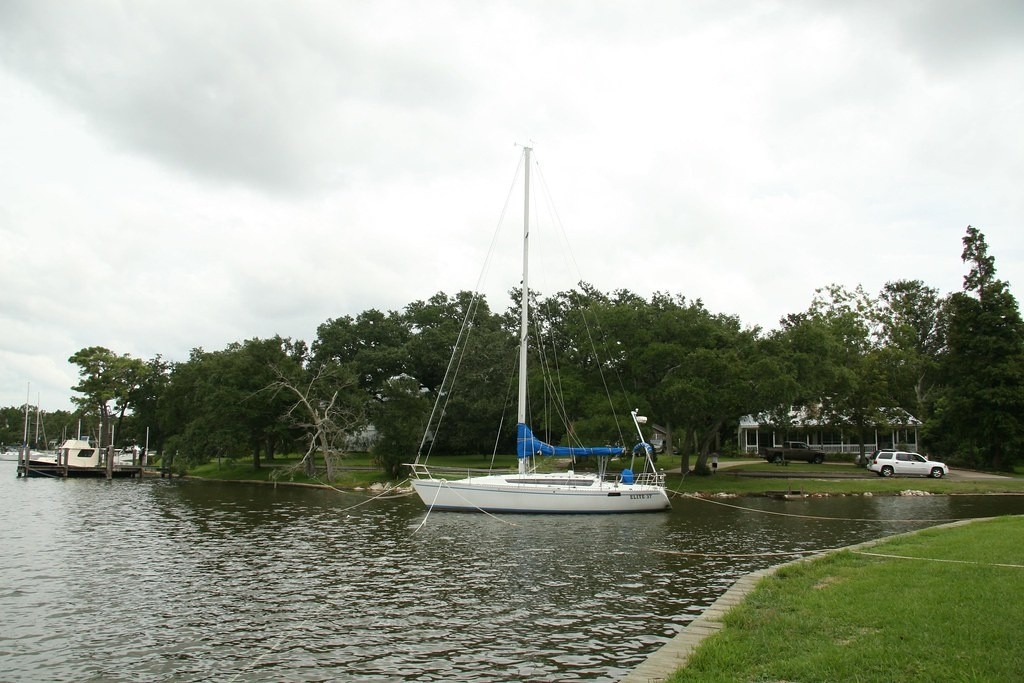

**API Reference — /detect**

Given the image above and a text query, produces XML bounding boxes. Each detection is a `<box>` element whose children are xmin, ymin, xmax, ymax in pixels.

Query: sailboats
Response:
<box><xmin>399</xmin><ymin>143</ymin><xmax>682</xmax><ymax>515</ymax></box>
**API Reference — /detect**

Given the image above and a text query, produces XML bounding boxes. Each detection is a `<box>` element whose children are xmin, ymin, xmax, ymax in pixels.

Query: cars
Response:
<box><xmin>855</xmin><ymin>453</ymin><xmax>872</xmax><ymax>466</ymax></box>
<box><xmin>649</xmin><ymin>440</ymin><xmax>667</xmax><ymax>456</ymax></box>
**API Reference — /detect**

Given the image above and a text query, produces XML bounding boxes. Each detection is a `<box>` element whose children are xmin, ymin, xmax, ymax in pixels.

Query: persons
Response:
<box><xmin>712</xmin><ymin>453</ymin><xmax>719</xmax><ymax>474</ymax></box>
<box><xmin>139</xmin><ymin>447</ymin><xmax>145</xmax><ymax>464</ymax></box>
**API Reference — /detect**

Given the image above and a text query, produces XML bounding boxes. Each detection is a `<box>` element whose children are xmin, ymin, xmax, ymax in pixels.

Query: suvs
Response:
<box><xmin>867</xmin><ymin>449</ymin><xmax>951</xmax><ymax>479</ymax></box>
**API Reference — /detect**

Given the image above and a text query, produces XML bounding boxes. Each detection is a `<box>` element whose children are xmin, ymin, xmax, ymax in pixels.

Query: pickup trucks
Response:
<box><xmin>763</xmin><ymin>440</ymin><xmax>827</xmax><ymax>465</ymax></box>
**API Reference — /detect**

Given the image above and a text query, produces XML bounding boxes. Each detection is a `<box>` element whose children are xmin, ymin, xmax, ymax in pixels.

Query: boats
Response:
<box><xmin>1</xmin><ymin>381</ymin><xmax>145</xmax><ymax>480</ymax></box>
